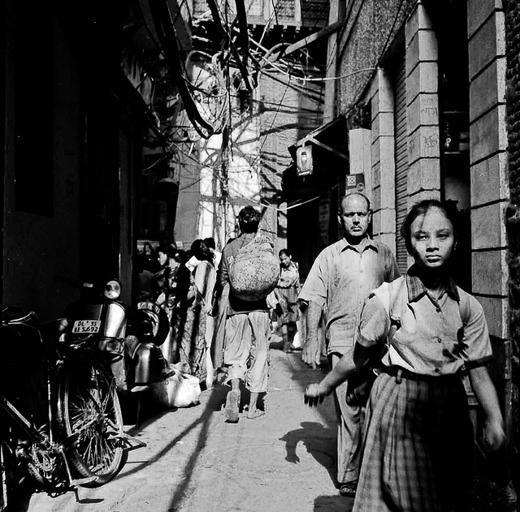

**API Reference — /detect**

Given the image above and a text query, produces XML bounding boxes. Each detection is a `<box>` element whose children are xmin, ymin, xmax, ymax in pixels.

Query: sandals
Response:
<box><xmin>340</xmin><ymin>481</ymin><xmax>357</xmax><ymax>495</ymax></box>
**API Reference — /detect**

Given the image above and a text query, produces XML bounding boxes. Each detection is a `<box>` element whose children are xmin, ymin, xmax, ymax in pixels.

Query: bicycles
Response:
<box><xmin>2</xmin><ymin>310</ymin><xmax>146</xmax><ymax>510</ymax></box>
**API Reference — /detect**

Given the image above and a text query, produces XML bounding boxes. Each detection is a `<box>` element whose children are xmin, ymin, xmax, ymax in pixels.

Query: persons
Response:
<box><xmin>304</xmin><ymin>200</ymin><xmax>506</xmax><ymax>512</ymax></box>
<box><xmin>298</xmin><ymin>193</ymin><xmax>399</xmax><ymax>496</ymax></box>
<box><xmin>157</xmin><ymin>206</ymin><xmax>302</xmax><ymax>422</ymax></box>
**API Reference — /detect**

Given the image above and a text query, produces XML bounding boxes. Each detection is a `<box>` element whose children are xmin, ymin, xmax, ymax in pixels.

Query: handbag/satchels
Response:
<box><xmin>226</xmin><ymin>234</ymin><xmax>280</xmax><ymax>301</ymax></box>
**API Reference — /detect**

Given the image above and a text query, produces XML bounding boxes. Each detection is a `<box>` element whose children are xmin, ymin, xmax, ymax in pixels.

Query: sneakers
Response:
<box><xmin>225</xmin><ymin>390</ymin><xmax>264</xmax><ymax>422</ymax></box>
